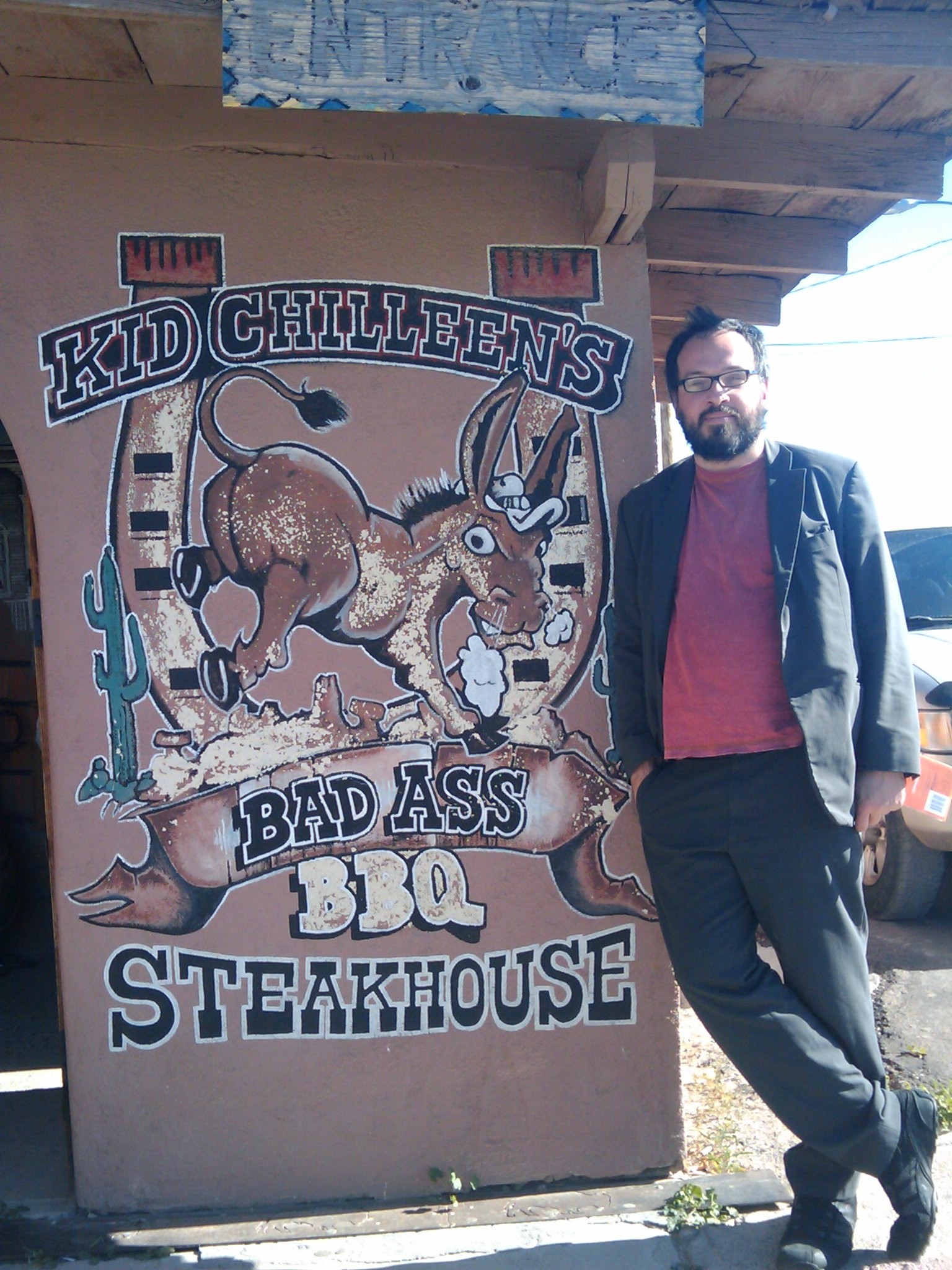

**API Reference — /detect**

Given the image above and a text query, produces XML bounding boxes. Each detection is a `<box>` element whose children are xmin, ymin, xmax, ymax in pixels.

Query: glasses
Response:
<box><xmin>675</xmin><ymin>370</ymin><xmax>757</xmax><ymax>394</ymax></box>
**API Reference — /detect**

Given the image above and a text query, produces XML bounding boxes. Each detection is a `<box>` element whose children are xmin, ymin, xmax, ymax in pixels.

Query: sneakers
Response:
<box><xmin>777</xmin><ymin>1195</ymin><xmax>858</xmax><ymax>1270</ymax></box>
<box><xmin>878</xmin><ymin>1090</ymin><xmax>938</xmax><ymax>1263</ymax></box>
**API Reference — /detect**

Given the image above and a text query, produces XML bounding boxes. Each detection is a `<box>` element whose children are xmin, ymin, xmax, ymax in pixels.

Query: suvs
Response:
<box><xmin>856</xmin><ymin>505</ymin><xmax>952</xmax><ymax>923</ymax></box>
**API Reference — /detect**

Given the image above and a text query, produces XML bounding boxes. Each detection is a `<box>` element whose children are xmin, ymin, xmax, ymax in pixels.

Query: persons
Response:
<box><xmin>601</xmin><ymin>304</ymin><xmax>942</xmax><ymax>1270</ymax></box>
